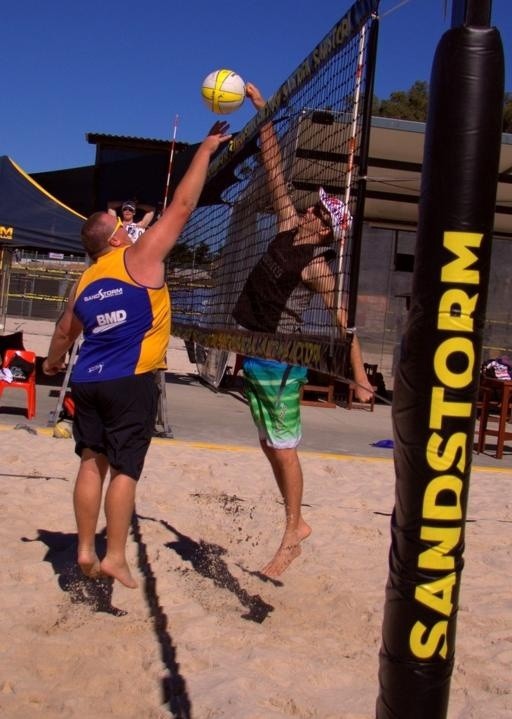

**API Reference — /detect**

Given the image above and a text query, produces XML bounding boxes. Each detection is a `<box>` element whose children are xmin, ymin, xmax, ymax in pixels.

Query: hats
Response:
<box><xmin>319</xmin><ymin>187</ymin><xmax>354</xmax><ymax>241</ymax></box>
<box><xmin>122</xmin><ymin>201</ymin><xmax>137</xmax><ymax>211</ymax></box>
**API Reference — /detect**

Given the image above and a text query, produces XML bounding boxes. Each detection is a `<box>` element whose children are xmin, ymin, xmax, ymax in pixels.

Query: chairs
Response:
<box><xmin>349</xmin><ymin>364</ymin><xmax>377</xmax><ymax>410</ymax></box>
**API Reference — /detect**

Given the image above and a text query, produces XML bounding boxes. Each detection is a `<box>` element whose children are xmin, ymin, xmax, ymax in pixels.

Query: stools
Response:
<box><xmin>0</xmin><ymin>349</ymin><xmax>36</xmax><ymax>421</ymax></box>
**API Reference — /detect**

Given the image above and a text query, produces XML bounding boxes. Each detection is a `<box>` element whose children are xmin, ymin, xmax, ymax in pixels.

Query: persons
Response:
<box><xmin>42</xmin><ymin>120</ymin><xmax>234</xmax><ymax>588</ymax></box>
<box><xmin>105</xmin><ymin>191</ymin><xmax>157</xmax><ymax>244</ymax></box>
<box><xmin>230</xmin><ymin>80</ymin><xmax>378</xmax><ymax>580</ymax></box>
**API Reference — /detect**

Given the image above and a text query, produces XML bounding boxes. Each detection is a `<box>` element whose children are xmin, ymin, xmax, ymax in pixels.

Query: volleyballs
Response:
<box><xmin>201</xmin><ymin>69</ymin><xmax>245</xmax><ymax>113</ymax></box>
<box><xmin>54</xmin><ymin>422</ymin><xmax>72</xmax><ymax>438</ymax></box>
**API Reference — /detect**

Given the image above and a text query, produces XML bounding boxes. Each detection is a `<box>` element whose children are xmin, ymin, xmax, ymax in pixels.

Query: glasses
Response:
<box><xmin>123</xmin><ymin>208</ymin><xmax>133</xmax><ymax>211</ymax></box>
<box><xmin>313</xmin><ymin>203</ymin><xmax>331</xmax><ymax>227</ymax></box>
<box><xmin>107</xmin><ymin>215</ymin><xmax>124</xmax><ymax>241</ymax></box>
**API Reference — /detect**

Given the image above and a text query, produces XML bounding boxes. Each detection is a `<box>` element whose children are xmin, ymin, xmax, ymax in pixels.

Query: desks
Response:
<box><xmin>473</xmin><ymin>377</ymin><xmax>512</xmax><ymax>459</ymax></box>
